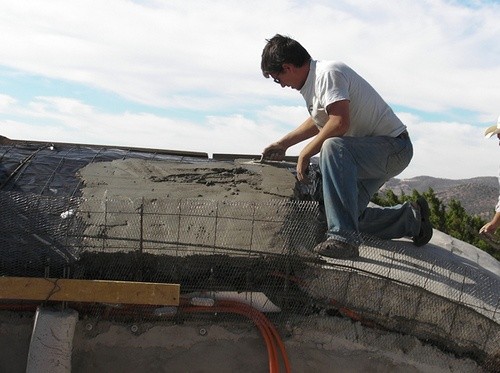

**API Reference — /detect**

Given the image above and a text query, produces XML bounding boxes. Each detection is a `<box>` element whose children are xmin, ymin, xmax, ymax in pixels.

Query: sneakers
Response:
<box><xmin>313</xmin><ymin>239</ymin><xmax>360</xmax><ymax>259</ymax></box>
<box><xmin>412</xmin><ymin>196</ymin><xmax>434</xmax><ymax>247</ymax></box>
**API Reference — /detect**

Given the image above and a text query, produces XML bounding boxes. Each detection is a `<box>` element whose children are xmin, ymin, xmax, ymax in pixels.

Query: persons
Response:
<box><xmin>478</xmin><ymin>124</ymin><xmax>500</xmax><ymax>234</ymax></box>
<box><xmin>258</xmin><ymin>34</ymin><xmax>433</xmax><ymax>259</ymax></box>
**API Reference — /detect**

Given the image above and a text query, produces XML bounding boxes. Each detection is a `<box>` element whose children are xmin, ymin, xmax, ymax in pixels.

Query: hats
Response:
<box><xmin>484</xmin><ymin>118</ymin><xmax>500</xmax><ymax>139</ymax></box>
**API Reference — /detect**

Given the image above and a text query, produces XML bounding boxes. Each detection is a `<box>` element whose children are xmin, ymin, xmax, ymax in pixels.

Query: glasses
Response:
<box><xmin>274</xmin><ymin>68</ymin><xmax>282</xmax><ymax>84</ymax></box>
